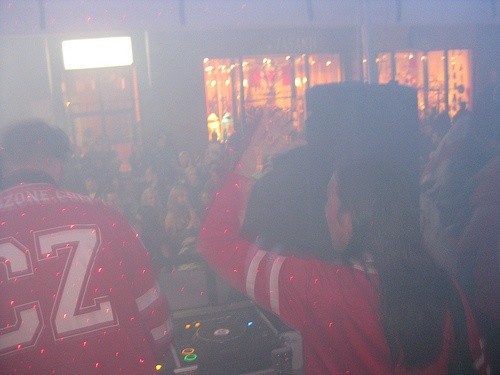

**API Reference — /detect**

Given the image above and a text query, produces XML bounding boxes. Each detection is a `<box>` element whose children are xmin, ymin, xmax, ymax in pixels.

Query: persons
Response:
<box><xmin>0</xmin><ymin>91</ymin><xmax>499</xmax><ymax>375</ymax></box>
